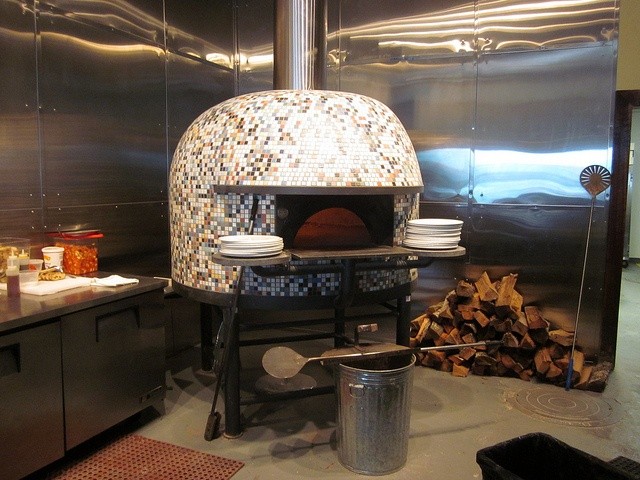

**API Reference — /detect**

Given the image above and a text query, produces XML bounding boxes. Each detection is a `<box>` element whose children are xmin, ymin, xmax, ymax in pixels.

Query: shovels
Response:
<box><xmin>565</xmin><ymin>166</ymin><xmax>612</xmax><ymax>392</ymax></box>
<box><xmin>261</xmin><ymin>341</ymin><xmax>505</xmax><ymax>379</ymax></box>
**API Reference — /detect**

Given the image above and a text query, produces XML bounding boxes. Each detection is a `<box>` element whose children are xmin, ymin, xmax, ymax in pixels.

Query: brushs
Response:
<box><xmin>203</xmin><ymin>196</ymin><xmax>260</xmax><ymax>442</ymax></box>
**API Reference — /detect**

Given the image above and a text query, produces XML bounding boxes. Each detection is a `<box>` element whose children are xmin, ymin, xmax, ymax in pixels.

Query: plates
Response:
<box><xmin>218</xmin><ymin>234</ymin><xmax>284</xmax><ymax>258</ymax></box>
<box><xmin>403</xmin><ymin>218</ymin><xmax>465</xmax><ymax>249</ymax></box>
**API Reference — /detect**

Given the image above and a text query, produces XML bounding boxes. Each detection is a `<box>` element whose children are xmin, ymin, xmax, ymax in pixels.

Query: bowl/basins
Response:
<box><xmin>29</xmin><ymin>260</ymin><xmax>42</xmax><ymax>271</ymax></box>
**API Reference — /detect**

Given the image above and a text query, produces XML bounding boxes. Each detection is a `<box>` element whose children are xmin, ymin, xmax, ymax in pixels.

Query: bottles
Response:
<box><xmin>42</xmin><ymin>247</ymin><xmax>64</xmax><ymax>269</ymax></box>
<box><xmin>6</xmin><ymin>260</ymin><xmax>20</xmax><ymax>297</ymax></box>
<box><xmin>19</xmin><ymin>249</ymin><xmax>29</xmax><ymax>270</ymax></box>
<box><xmin>7</xmin><ymin>250</ymin><xmax>18</xmax><ymax>267</ymax></box>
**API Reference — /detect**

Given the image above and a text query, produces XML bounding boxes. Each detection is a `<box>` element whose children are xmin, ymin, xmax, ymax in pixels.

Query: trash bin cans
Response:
<box><xmin>473</xmin><ymin>430</ymin><xmax>637</xmax><ymax>475</ymax></box>
